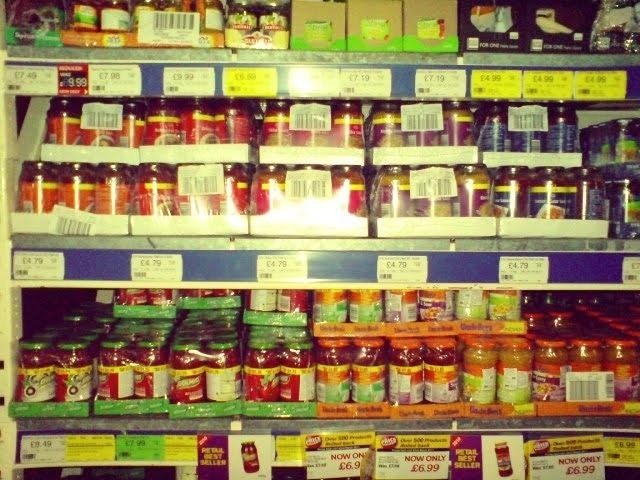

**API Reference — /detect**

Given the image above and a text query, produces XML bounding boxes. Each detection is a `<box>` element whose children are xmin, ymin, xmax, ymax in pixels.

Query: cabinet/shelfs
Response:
<box><xmin>0</xmin><ymin>0</ymin><xmax>640</xmax><ymax>480</ymax></box>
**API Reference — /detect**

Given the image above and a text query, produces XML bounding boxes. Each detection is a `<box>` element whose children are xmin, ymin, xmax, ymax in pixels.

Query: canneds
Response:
<box><xmin>351</xmin><ymin>337</ymin><xmax>389</xmax><ymax>405</ymax></box>
<box><xmin>249</xmin><ymin>165</ymin><xmax>286</xmax><ymax>213</ymax></box>
<box><xmin>240</xmin><ymin>440</ymin><xmax>259</xmax><ymax>473</ymax></box>
<box><xmin>312</xmin><ymin>289</ymin><xmax>347</xmax><ymax>323</ymax></box>
<box><xmin>113</xmin><ymin>101</ymin><xmax>146</xmax><ymax>146</ymax></box>
<box><xmin>182</xmin><ymin>99</ymin><xmax>216</xmax><ymax>143</ymax></box>
<box><xmin>407</xmin><ymin>101</ymin><xmax>440</xmax><ymax>146</ymax></box>
<box><xmin>17</xmin><ymin>309</ymin><xmax>113</xmax><ymax>401</ymax></box>
<box><xmin>455</xmin><ymin>165</ymin><xmax>494</xmax><ymax>216</ymax></box>
<box><xmin>331</xmin><ymin>165</ymin><xmax>367</xmax><ymax>215</ymax></box>
<box><xmin>498</xmin><ymin>338</ymin><xmax>533</xmax><ymax>404</ymax></box>
<box><xmin>99</xmin><ymin>0</ymin><xmax>131</xmax><ymax>32</ymax></box>
<box><xmin>477</xmin><ymin>98</ymin><xmax>512</xmax><ymax>152</ymax></box>
<box><xmin>441</xmin><ymin>101</ymin><xmax>475</xmax><ymax>144</ymax></box>
<box><xmin>276</xmin><ymin>289</ymin><xmax>309</xmax><ymax>312</ymax></box>
<box><xmin>495</xmin><ymin>442</ymin><xmax>513</xmax><ymax>478</ymax></box>
<box><xmin>117</xmin><ymin>287</ymin><xmax>146</xmax><ymax>306</ymax></box>
<box><xmin>174</xmin><ymin>163</ymin><xmax>211</xmax><ymax>216</ymax></box>
<box><xmin>217</xmin><ymin>97</ymin><xmax>255</xmax><ymax>143</ymax></box>
<box><xmin>581</xmin><ymin>117</ymin><xmax>640</xmax><ymax>181</ymax></box>
<box><xmin>63</xmin><ymin>1</ymin><xmax>99</xmax><ymax>32</ymax></box>
<box><xmin>419</xmin><ymin>290</ymin><xmax>455</xmax><ymax>321</ymax></box>
<box><xmin>523</xmin><ymin>297</ymin><xmax>640</xmax><ymax>402</ymax></box>
<box><xmin>425</xmin><ymin>334</ymin><xmax>457</xmax><ymax>406</ymax></box>
<box><xmin>193</xmin><ymin>0</ymin><xmax>225</xmax><ymax>32</ymax></box>
<box><xmin>177</xmin><ymin>289</ymin><xmax>212</xmax><ymax>298</ymax></box>
<box><xmin>132</xmin><ymin>163</ymin><xmax>174</xmax><ymax>215</ymax></box>
<box><xmin>494</xmin><ymin>166</ymin><xmax>532</xmax><ymax>216</ymax></box>
<box><xmin>291</xmin><ymin>165</ymin><xmax>324</xmax><ymax>172</ymax></box>
<box><xmin>212</xmin><ymin>165</ymin><xmax>249</xmax><ymax>215</ymax></box>
<box><xmin>212</xmin><ymin>289</ymin><xmax>241</xmax><ymax>298</ymax></box>
<box><xmin>605</xmin><ymin>180</ymin><xmax>640</xmax><ymax>239</ymax></box>
<box><xmin>82</xmin><ymin>97</ymin><xmax>113</xmax><ymax>146</ymax></box>
<box><xmin>166</xmin><ymin>309</ymin><xmax>242</xmax><ymax>403</ymax></box>
<box><xmin>315</xmin><ymin>337</ymin><xmax>351</xmax><ymax>403</ymax></box>
<box><xmin>295</xmin><ymin>100</ymin><xmax>328</xmax><ymax>147</ymax></box>
<box><xmin>370</xmin><ymin>103</ymin><xmax>406</xmax><ymax>146</ymax></box>
<box><xmin>455</xmin><ymin>290</ymin><xmax>489</xmax><ymax>322</ymax></box>
<box><xmin>158</xmin><ymin>1</ymin><xmax>189</xmax><ymax>30</ymax></box>
<box><xmin>532</xmin><ymin>167</ymin><xmax>569</xmax><ymax>217</ymax></box>
<box><xmin>243</xmin><ymin>326</ymin><xmax>315</xmax><ymax>403</ymax></box>
<box><xmin>57</xmin><ymin>162</ymin><xmax>94</xmax><ymax>215</ymax></box>
<box><xmin>513</xmin><ymin>103</ymin><xmax>544</xmax><ymax>153</ymax></box>
<box><xmin>95</xmin><ymin>163</ymin><xmax>131</xmax><ymax>215</ymax></box>
<box><xmin>570</xmin><ymin>168</ymin><xmax>604</xmax><ymax>219</ymax></box>
<box><xmin>46</xmin><ymin>98</ymin><xmax>82</xmax><ymax>146</ymax></box>
<box><xmin>383</xmin><ymin>290</ymin><xmax>419</xmax><ymax>323</ymax></box>
<box><xmin>376</xmin><ymin>164</ymin><xmax>412</xmax><ymax>217</ymax></box>
<box><xmin>329</xmin><ymin>99</ymin><xmax>365</xmax><ymax>147</ymax></box>
<box><xmin>146</xmin><ymin>289</ymin><xmax>175</xmax><ymax>305</ymax></box>
<box><xmin>489</xmin><ymin>292</ymin><xmax>522</xmax><ymax>321</ymax></box>
<box><xmin>227</xmin><ymin>0</ymin><xmax>257</xmax><ymax>28</ymax></box>
<box><xmin>389</xmin><ymin>337</ymin><xmax>425</xmax><ymax>406</ymax></box>
<box><xmin>146</xmin><ymin>100</ymin><xmax>182</xmax><ymax>146</ymax></box>
<box><xmin>258</xmin><ymin>0</ymin><xmax>290</xmax><ymax>28</ymax></box>
<box><xmin>132</xmin><ymin>0</ymin><xmax>158</xmax><ymax>31</ymax></box>
<box><xmin>544</xmin><ymin>100</ymin><xmax>579</xmax><ymax>153</ymax></box>
<box><xmin>243</xmin><ymin>290</ymin><xmax>277</xmax><ymax>311</ymax></box>
<box><xmin>262</xmin><ymin>99</ymin><xmax>294</xmax><ymax>147</ymax></box>
<box><xmin>458</xmin><ymin>336</ymin><xmax>499</xmax><ymax>403</ymax></box>
<box><xmin>100</xmin><ymin>319</ymin><xmax>177</xmax><ymax>401</ymax></box>
<box><xmin>347</xmin><ymin>289</ymin><xmax>383</xmax><ymax>323</ymax></box>
<box><xmin>415</xmin><ymin>164</ymin><xmax>453</xmax><ymax>216</ymax></box>
<box><xmin>19</xmin><ymin>161</ymin><xmax>57</xmax><ymax>213</ymax></box>
